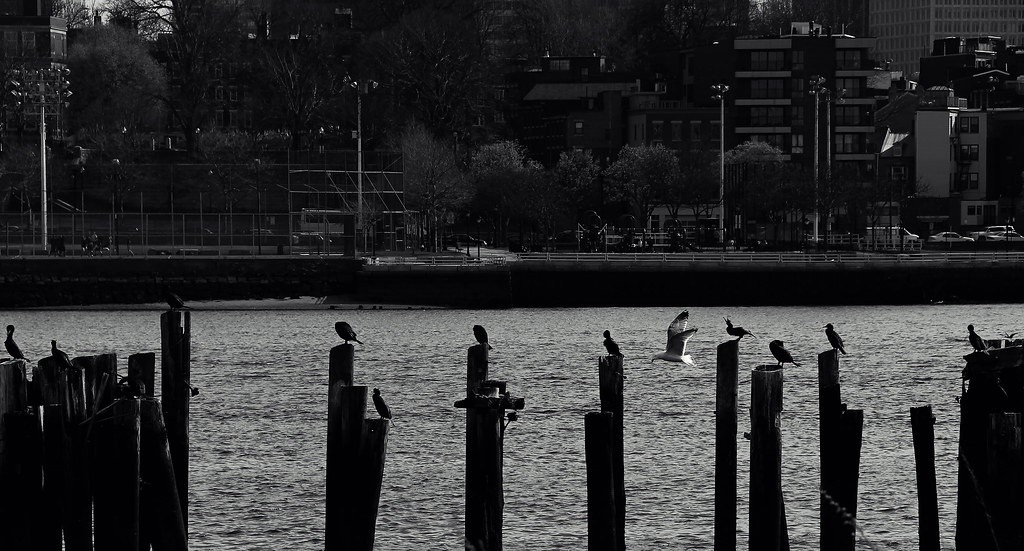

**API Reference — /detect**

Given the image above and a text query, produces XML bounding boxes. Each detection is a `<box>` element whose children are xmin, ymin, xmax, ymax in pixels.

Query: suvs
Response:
<box><xmin>976</xmin><ymin>225</ymin><xmax>1015</xmax><ymax>239</ymax></box>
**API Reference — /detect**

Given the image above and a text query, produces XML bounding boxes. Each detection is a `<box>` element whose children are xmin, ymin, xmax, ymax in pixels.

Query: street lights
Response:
<box><xmin>111</xmin><ymin>159</ymin><xmax>121</xmax><ymax>254</ymax></box>
<box><xmin>346</xmin><ymin>79</ymin><xmax>381</xmax><ymax>225</ymax></box>
<box><xmin>817</xmin><ymin>84</ymin><xmax>846</xmax><ymax>244</ymax></box>
<box><xmin>709</xmin><ymin>83</ymin><xmax>729</xmax><ymax>244</ymax></box>
<box><xmin>10</xmin><ymin>63</ymin><xmax>71</xmax><ymax>253</ymax></box>
<box><xmin>808</xmin><ymin>74</ymin><xmax>828</xmax><ymax>245</ymax></box>
<box><xmin>254</xmin><ymin>157</ymin><xmax>262</xmax><ymax>255</ymax></box>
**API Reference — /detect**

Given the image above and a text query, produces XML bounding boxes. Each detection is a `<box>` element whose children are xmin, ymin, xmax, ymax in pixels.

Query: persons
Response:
<box><xmin>87</xmin><ymin>231</ymin><xmax>98</xmax><ymax>240</ymax></box>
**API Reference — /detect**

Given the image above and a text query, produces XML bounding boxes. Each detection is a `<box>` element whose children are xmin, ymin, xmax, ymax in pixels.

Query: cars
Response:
<box><xmin>986</xmin><ymin>230</ymin><xmax>1024</xmax><ymax>241</ymax></box>
<box><xmin>444</xmin><ymin>234</ymin><xmax>489</xmax><ymax>248</ymax></box>
<box><xmin>927</xmin><ymin>231</ymin><xmax>977</xmax><ymax>244</ymax></box>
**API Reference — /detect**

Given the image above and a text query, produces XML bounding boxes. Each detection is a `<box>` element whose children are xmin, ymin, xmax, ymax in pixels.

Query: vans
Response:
<box><xmin>864</xmin><ymin>227</ymin><xmax>922</xmax><ymax>248</ymax></box>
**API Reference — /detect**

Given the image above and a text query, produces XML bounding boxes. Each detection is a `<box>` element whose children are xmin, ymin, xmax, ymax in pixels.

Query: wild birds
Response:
<box><xmin>50</xmin><ymin>340</ymin><xmax>75</xmax><ymax>368</ymax></box>
<box><xmin>473</xmin><ymin>323</ymin><xmax>493</xmax><ymax>351</ymax></box>
<box><xmin>1003</xmin><ymin>331</ymin><xmax>1018</xmax><ymax>339</ymax></box>
<box><xmin>165</xmin><ymin>284</ymin><xmax>195</xmax><ymax>312</ymax></box>
<box><xmin>822</xmin><ymin>321</ymin><xmax>847</xmax><ymax>356</ymax></box>
<box><xmin>651</xmin><ymin>309</ymin><xmax>699</xmax><ymax>368</ymax></box>
<box><xmin>372</xmin><ymin>387</ymin><xmax>396</xmax><ymax>427</ymax></box>
<box><xmin>768</xmin><ymin>339</ymin><xmax>802</xmax><ymax>367</ymax></box>
<box><xmin>3</xmin><ymin>325</ymin><xmax>30</xmax><ymax>363</ymax></box>
<box><xmin>722</xmin><ymin>316</ymin><xmax>757</xmax><ymax>341</ymax></box>
<box><xmin>603</xmin><ymin>330</ymin><xmax>626</xmax><ymax>359</ymax></box>
<box><xmin>967</xmin><ymin>324</ymin><xmax>991</xmax><ymax>356</ymax></box>
<box><xmin>334</xmin><ymin>321</ymin><xmax>364</xmax><ymax>346</ymax></box>
